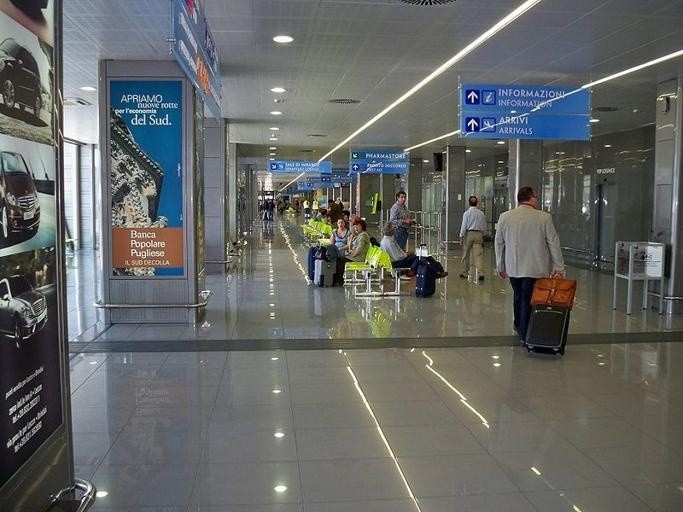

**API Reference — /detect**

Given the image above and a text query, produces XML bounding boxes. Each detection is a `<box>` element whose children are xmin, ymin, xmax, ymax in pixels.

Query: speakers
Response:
<box><xmin>433</xmin><ymin>153</ymin><xmax>442</xmax><ymax>172</ymax></box>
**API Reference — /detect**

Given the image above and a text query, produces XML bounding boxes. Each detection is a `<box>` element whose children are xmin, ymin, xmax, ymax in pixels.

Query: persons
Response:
<box><xmin>259</xmin><ymin>196</ymin><xmax>285</xmax><ymax>221</ymax></box>
<box><xmin>495</xmin><ymin>186</ymin><xmax>566</xmax><ymax>346</ymax></box>
<box><xmin>459</xmin><ymin>195</ymin><xmax>487</xmax><ymax>281</ymax></box>
<box><xmin>389</xmin><ymin>191</ymin><xmax>416</xmax><ymax>251</ymax></box>
<box><xmin>295</xmin><ymin>198</ymin><xmax>370</xmax><ymax>286</ymax></box>
<box><xmin>380</xmin><ymin>222</ymin><xmax>448</xmax><ymax>279</ymax></box>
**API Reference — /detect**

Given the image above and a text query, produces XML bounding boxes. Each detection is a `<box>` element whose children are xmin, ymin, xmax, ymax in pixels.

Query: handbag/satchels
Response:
<box><xmin>530</xmin><ymin>278</ymin><xmax>576</xmax><ymax>309</ymax></box>
<box><xmin>325</xmin><ymin>244</ymin><xmax>339</xmax><ymax>261</ymax></box>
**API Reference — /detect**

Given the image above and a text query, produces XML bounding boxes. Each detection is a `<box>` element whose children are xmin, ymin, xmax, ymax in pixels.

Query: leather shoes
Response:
<box><xmin>459</xmin><ymin>274</ymin><xmax>485</xmax><ymax>281</ymax></box>
<box><xmin>436</xmin><ymin>271</ymin><xmax>448</xmax><ymax>278</ymax></box>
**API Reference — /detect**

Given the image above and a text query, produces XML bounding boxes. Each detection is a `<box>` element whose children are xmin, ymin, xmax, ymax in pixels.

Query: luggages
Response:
<box><xmin>308</xmin><ymin>246</ymin><xmax>337</xmax><ymax>288</ymax></box>
<box><xmin>416</xmin><ymin>243</ymin><xmax>435</xmax><ymax>298</ymax></box>
<box><xmin>525</xmin><ymin>271</ymin><xmax>570</xmax><ymax>357</ymax></box>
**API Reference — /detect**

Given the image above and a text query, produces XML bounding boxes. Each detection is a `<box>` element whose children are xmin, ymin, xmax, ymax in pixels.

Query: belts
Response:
<box><xmin>467</xmin><ymin>230</ymin><xmax>479</xmax><ymax>231</ymax></box>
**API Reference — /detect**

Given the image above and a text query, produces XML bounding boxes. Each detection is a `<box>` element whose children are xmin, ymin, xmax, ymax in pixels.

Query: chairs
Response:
<box><xmin>300</xmin><ymin>218</ymin><xmax>412</xmax><ymax>299</ymax></box>
